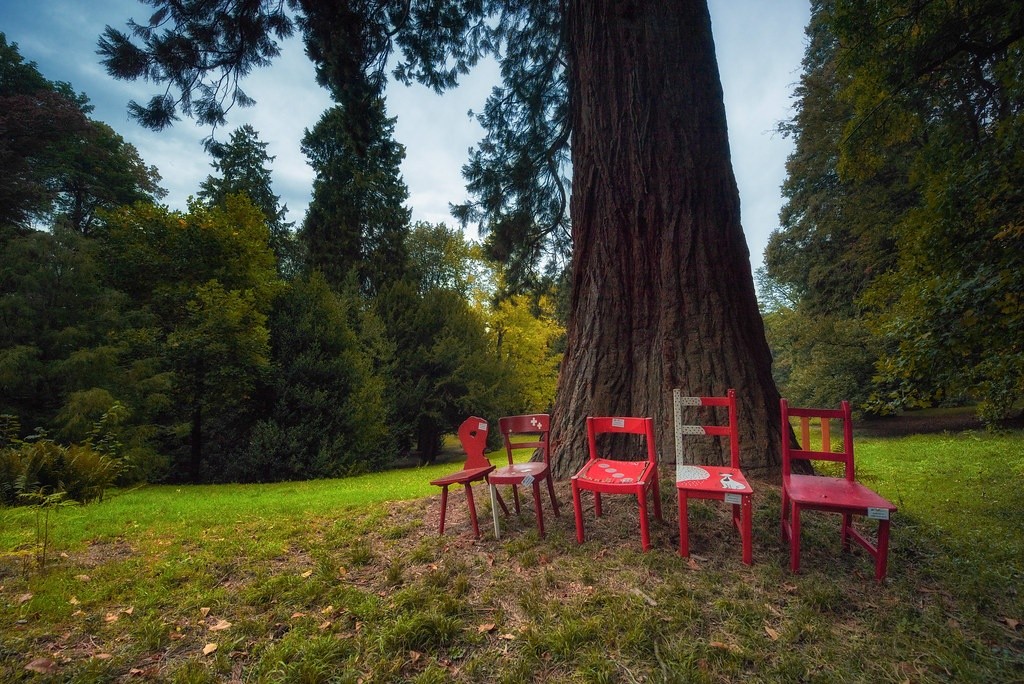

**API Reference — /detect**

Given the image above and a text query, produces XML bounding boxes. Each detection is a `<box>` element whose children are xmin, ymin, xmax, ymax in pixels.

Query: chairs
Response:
<box><xmin>779</xmin><ymin>398</ymin><xmax>898</xmax><ymax>583</ymax></box>
<box><xmin>672</xmin><ymin>388</ymin><xmax>754</xmax><ymax>567</ymax></box>
<box><xmin>430</xmin><ymin>416</ymin><xmax>509</xmax><ymax>539</ymax></box>
<box><xmin>571</xmin><ymin>416</ymin><xmax>663</xmax><ymax>553</ymax></box>
<box><xmin>487</xmin><ymin>414</ymin><xmax>560</xmax><ymax>540</ymax></box>
